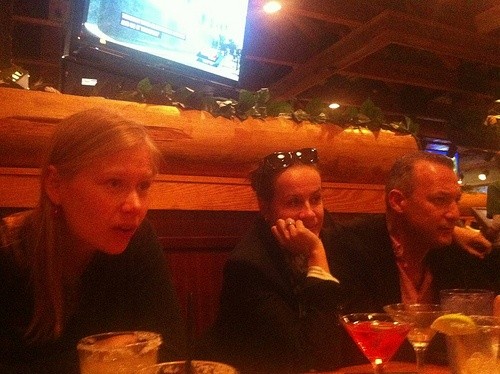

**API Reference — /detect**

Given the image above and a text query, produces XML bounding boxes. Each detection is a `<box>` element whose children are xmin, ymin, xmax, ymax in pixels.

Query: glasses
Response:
<box><xmin>264</xmin><ymin>147</ymin><xmax>317</xmax><ymax>170</ymax></box>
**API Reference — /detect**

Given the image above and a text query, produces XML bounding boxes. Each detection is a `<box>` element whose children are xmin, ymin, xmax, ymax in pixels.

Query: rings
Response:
<box><xmin>287</xmin><ymin>222</ymin><xmax>295</xmax><ymax>227</ymax></box>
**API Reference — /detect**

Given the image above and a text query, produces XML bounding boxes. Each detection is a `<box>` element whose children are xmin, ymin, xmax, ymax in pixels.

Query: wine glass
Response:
<box><xmin>340</xmin><ymin>313</ymin><xmax>414</xmax><ymax>374</ymax></box>
<box><xmin>383</xmin><ymin>303</ymin><xmax>459</xmax><ymax>374</ymax></box>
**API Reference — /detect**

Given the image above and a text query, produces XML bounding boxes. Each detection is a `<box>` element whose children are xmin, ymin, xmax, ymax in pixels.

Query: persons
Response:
<box><xmin>0</xmin><ymin>109</ymin><xmax>174</xmax><ymax>374</ymax></box>
<box><xmin>194</xmin><ymin>147</ymin><xmax>500</xmax><ymax>374</ymax></box>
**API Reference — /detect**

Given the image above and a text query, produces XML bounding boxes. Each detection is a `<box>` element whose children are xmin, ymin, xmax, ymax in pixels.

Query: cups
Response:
<box><xmin>440</xmin><ymin>288</ymin><xmax>500</xmax><ymax>374</ymax></box>
<box><xmin>76</xmin><ymin>330</ymin><xmax>160</xmax><ymax>374</ymax></box>
<box><xmin>134</xmin><ymin>360</ymin><xmax>239</xmax><ymax>374</ymax></box>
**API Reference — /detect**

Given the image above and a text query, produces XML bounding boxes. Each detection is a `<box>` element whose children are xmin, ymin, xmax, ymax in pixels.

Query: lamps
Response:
<box><xmin>446</xmin><ymin>144</ymin><xmax>496</xmax><ymax>184</ymax></box>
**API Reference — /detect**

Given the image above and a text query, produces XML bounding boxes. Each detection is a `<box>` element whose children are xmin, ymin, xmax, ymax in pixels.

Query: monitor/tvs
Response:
<box><xmin>62</xmin><ymin>0</ymin><xmax>252</xmax><ymax>96</ymax></box>
